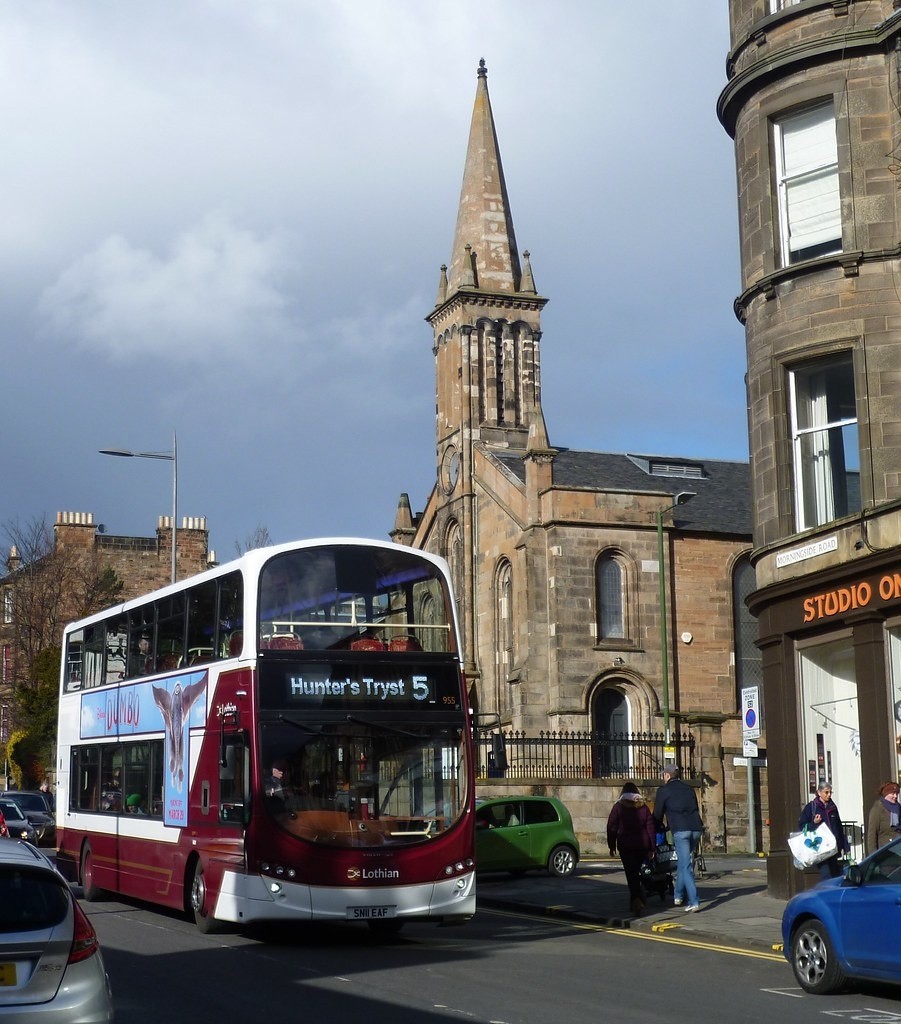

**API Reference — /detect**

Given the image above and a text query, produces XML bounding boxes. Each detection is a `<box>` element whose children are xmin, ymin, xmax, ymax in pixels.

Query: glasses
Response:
<box><xmin>821</xmin><ymin>790</ymin><xmax>833</xmax><ymax>794</ymax></box>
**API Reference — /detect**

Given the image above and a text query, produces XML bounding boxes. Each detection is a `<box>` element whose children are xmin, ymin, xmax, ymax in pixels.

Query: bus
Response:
<box><xmin>55</xmin><ymin>537</ymin><xmax>508</xmax><ymax>934</ymax></box>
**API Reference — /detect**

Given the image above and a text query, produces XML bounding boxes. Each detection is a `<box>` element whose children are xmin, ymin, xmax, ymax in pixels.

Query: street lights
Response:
<box><xmin>98</xmin><ymin>450</ymin><xmax>178</xmax><ymax>585</ymax></box>
<box><xmin>657</xmin><ymin>491</ymin><xmax>697</xmax><ymax>766</ymax></box>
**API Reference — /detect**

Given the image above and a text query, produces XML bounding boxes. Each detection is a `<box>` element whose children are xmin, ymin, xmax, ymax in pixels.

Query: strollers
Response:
<box><xmin>638</xmin><ymin>827</ymin><xmax>679</xmax><ymax>904</ymax></box>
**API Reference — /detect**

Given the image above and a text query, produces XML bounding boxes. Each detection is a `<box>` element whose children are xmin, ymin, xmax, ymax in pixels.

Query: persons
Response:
<box><xmin>101</xmin><ymin>766</ymin><xmax>162</xmax><ymax>816</ymax></box>
<box><xmin>652</xmin><ymin>764</ymin><xmax>706</xmax><ymax>912</ymax></box>
<box><xmin>265</xmin><ymin>756</ymin><xmax>299</xmax><ymax>803</ymax></box>
<box><xmin>865</xmin><ymin>779</ymin><xmax>901</xmax><ymax>877</ymax></box>
<box><xmin>40</xmin><ymin>785</ymin><xmax>52</xmax><ymax>808</ymax></box>
<box><xmin>800</xmin><ymin>779</ymin><xmax>850</xmax><ymax>883</ymax></box>
<box><xmin>131</xmin><ymin>634</ymin><xmax>161</xmax><ymax>676</ymax></box>
<box><xmin>605</xmin><ymin>780</ymin><xmax>659</xmax><ymax>913</ymax></box>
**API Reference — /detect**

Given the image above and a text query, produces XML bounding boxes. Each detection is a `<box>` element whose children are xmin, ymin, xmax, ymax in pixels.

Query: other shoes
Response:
<box><xmin>674</xmin><ymin>899</ymin><xmax>683</xmax><ymax>904</ymax></box>
<box><xmin>634</xmin><ymin>897</ymin><xmax>644</xmax><ymax>917</ymax></box>
<box><xmin>684</xmin><ymin>905</ymin><xmax>700</xmax><ymax>912</ymax></box>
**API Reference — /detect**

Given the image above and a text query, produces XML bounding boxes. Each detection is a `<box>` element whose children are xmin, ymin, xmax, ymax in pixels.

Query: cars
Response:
<box><xmin>0</xmin><ymin>833</ymin><xmax>115</xmax><ymax>1024</ymax></box>
<box><xmin>424</xmin><ymin>795</ymin><xmax>580</xmax><ymax>877</ymax></box>
<box><xmin>0</xmin><ymin>798</ymin><xmax>40</xmax><ymax>848</ymax></box>
<box><xmin>782</xmin><ymin>836</ymin><xmax>901</xmax><ymax>996</ymax></box>
<box><xmin>1</xmin><ymin>791</ymin><xmax>56</xmax><ymax>842</ymax></box>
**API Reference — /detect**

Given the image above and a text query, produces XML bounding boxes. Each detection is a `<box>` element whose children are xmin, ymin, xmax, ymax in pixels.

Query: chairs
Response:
<box><xmin>349</xmin><ymin>633</ymin><xmax>423</xmax><ymax>652</ymax></box>
<box><xmin>100</xmin><ymin>791</ymin><xmax>163</xmax><ymax>815</ymax></box>
<box><xmin>144</xmin><ymin>629</ymin><xmax>305</xmax><ymax>676</ymax></box>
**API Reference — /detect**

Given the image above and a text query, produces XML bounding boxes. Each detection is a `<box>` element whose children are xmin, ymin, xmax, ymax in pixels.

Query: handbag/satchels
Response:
<box><xmin>788</xmin><ymin>822</ymin><xmax>837</xmax><ymax>870</ymax></box>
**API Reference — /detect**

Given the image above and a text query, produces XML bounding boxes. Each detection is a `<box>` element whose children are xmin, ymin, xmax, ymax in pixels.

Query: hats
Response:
<box><xmin>879</xmin><ymin>782</ymin><xmax>900</xmax><ymax>798</ymax></box>
<box><xmin>127</xmin><ymin>794</ymin><xmax>141</xmax><ymax>806</ymax></box>
<box><xmin>662</xmin><ymin>764</ymin><xmax>679</xmax><ymax>773</ymax></box>
